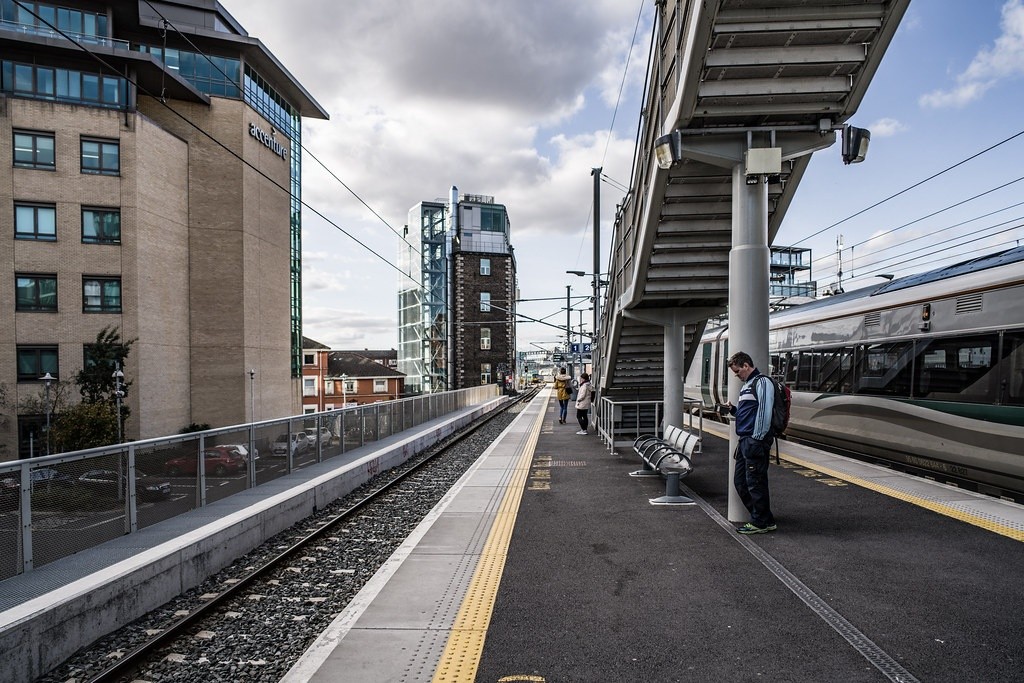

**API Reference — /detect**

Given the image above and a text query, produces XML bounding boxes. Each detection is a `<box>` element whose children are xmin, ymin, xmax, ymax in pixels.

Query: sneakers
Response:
<box><xmin>767</xmin><ymin>522</ymin><xmax>777</xmax><ymax>531</ymax></box>
<box><xmin>737</xmin><ymin>521</ymin><xmax>768</xmax><ymax>534</ymax></box>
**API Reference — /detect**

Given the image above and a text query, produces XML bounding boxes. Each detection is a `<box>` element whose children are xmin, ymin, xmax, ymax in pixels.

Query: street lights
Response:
<box><xmin>561</xmin><ymin>307</ymin><xmax>595</xmax><ymax>386</ymax></box>
<box><xmin>38</xmin><ymin>373</ymin><xmax>57</xmax><ymax>456</ymax></box>
<box><xmin>248</xmin><ymin>369</ymin><xmax>257</xmax><ymax>489</ymax></box>
<box><xmin>112</xmin><ymin>362</ymin><xmax>127</xmax><ymax>501</ymax></box>
<box><xmin>340</xmin><ymin>373</ymin><xmax>348</xmax><ymax>454</ymax></box>
<box><xmin>566</xmin><ymin>270</ymin><xmax>611</xmax><ymax>342</ymax></box>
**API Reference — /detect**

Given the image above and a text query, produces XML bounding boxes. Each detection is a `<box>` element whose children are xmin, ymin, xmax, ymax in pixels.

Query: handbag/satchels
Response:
<box><xmin>565</xmin><ymin>379</ymin><xmax>573</xmax><ymax>395</ymax></box>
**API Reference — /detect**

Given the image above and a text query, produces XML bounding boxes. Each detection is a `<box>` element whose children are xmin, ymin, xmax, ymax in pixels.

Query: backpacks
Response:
<box><xmin>751</xmin><ymin>374</ymin><xmax>792</xmax><ymax>435</ymax></box>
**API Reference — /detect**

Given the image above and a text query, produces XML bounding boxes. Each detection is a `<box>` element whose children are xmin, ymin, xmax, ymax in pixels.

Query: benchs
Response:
<box><xmin>628</xmin><ymin>424</ymin><xmax>702</xmax><ymax>506</ymax></box>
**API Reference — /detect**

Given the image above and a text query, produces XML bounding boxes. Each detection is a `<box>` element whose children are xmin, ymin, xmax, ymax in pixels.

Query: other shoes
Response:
<box><xmin>563</xmin><ymin>418</ymin><xmax>566</xmax><ymax>423</ymax></box>
<box><xmin>559</xmin><ymin>418</ymin><xmax>563</xmax><ymax>424</ymax></box>
<box><xmin>576</xmin><ymin>428</ymin><xmax>588</xmax><ymax>435</ymax></box>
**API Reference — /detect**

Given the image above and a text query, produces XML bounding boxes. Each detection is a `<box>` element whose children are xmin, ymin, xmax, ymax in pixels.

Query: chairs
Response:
<box><xmin>919</xmin><ymin>368</ymin><xmax>988</xmax><ymax>399</ymax></box>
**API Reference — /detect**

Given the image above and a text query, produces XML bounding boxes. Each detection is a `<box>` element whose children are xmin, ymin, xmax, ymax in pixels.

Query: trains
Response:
<box><xmin>684</xmin><ymin>243</ymin><xmax>1024</xmax><ymax>500</ymax></box>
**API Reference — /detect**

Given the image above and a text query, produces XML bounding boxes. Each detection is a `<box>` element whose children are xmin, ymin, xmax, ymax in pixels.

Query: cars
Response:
<box><xmin>166</xmin><ymin>446</ymin><xmax>244</xmax><ymax>478</ymax></box>
<box><xmin>303</xmin><ymin>427</ymin><xmax>334</xmax><ymax>449</ymax></box>
<box><xmin>271</xmin><ymin>432</ymin><xmax>310</xmax><ymax>459</ymax></box>
<box><xmin>78</xmin><ymin>467</ymin><xmax>171</xmax><ymax>503</ymax></box>
<box><xmin>0</xmin><ymin>467</ymin><xmax>79</xmax><ymax>510</ymax></box>
<box><xmin>213</xmin><ymin>444</ymin><xmax>261</xmax><ymax>469</ymax></box>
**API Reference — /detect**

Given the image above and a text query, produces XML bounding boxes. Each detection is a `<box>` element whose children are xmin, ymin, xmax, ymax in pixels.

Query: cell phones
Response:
<box><xmin>717</xmin><ymin>403</ymin><xmax>730</xmax><ymax>408</ymax></box>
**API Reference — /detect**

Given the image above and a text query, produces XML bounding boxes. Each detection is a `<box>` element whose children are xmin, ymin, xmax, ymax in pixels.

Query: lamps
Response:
<box><xmin>843</xmin><ymin>125</ymin><xmax>871</xmax><ymax>165</ymax></box>
<box><xmin>746</xmin><ymin>176</ymin><xmax>759</xmax><ymax>185</ymax></box>
<box><xmin>653</xmin><ymin>131</ymin><xmax>683</xmax><ymax>169</ymax></box>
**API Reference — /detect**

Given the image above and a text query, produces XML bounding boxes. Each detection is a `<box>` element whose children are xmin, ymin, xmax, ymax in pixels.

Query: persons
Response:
<box><xmin>554</xmin><ymin>367</ymin><xmax>572</xmax><ymax>424</ymax></box>
<box><xmin>575</xmin><ymin>373</ymin><xmax>592</xmax><ymax>435</ymax></box>
<box><xmin>721</xmin><ymin>351</ymin><xmax>777</xmax><ymax>534</ymax></box>
